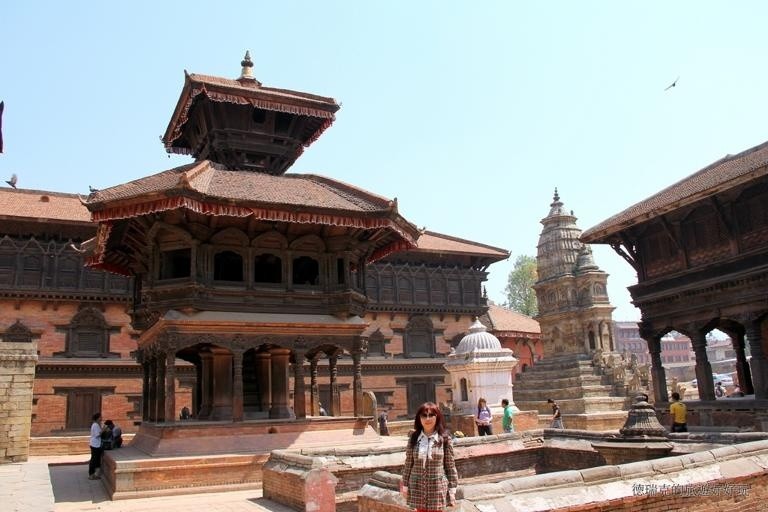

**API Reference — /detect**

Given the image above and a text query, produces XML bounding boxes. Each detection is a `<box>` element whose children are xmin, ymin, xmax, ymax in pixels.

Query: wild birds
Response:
<box><xmin>665</xmin><ymin>78</ymin><xmax>679</xmax><ymax>91</ymax></box>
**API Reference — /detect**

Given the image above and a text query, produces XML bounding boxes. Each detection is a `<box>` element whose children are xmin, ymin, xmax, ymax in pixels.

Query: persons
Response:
<box><xmin>98</xmin><ymin>420</ymin><xmax>122</xmax><ymax>454</ymax></box>
<box><xmin>547</xmin><ymin>399</ymin><xmax>564</xmax><ymax>430</ymax></box>
<box><xmin>668</xmin><ymin>392</ymin><xmax>687</xmax><ymax>432</ymax></box>
<box><xmin>87</xmin><ymin>413</ymin><xmax>108</xmax><ymax>481</ymax></box>
<box><xmin>318</xmin><ymin>402</ymin><xmax>326</xmax><ymax>416</ymax></box>
<box><xmin>377</xmin><ymin>410</ymin><xmax>389</xmax><ymax>436</ymax></box>
<box><xmin>714</xmin><ymin>382</ymin><xmax>739</xmax><ymax>398</ymax></box>
<box><xmin>500</xmin><ymin>399</ymin><xmax>514</xmax><ymax>434</ymax></box>
<box><xmin>473</xmin><ymin>397</ymin><xmax>493</xmax><ymax>436</ymax></box>
<box><xmin>400</xmin><ymin>401</ymin><xmax>457</xmax><ymax>512</ymax></box>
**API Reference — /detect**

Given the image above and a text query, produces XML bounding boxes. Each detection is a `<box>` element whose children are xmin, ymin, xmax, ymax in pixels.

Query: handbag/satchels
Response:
<box><xmin>102</xmin><ymin>441</ymin><xmax>112</xmax><ymax>450</ymax></box>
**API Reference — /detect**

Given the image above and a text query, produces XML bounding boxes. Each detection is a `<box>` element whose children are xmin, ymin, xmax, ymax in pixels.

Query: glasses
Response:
<box><xmin>420</xmin><ymin>413</ymin><xmax>436</xmax><ymax>418</ymax></box>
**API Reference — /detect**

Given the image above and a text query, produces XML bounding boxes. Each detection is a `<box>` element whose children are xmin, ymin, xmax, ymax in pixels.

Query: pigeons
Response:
<box><xmin>89</xmin><ymin>186</ymin><xmax>98</xmax><ymax>192</ymax></box>
<box><xmin>5</xmin><ymin>175</ymin><xmax>18</xmax><ymax>189</ymax></box>
